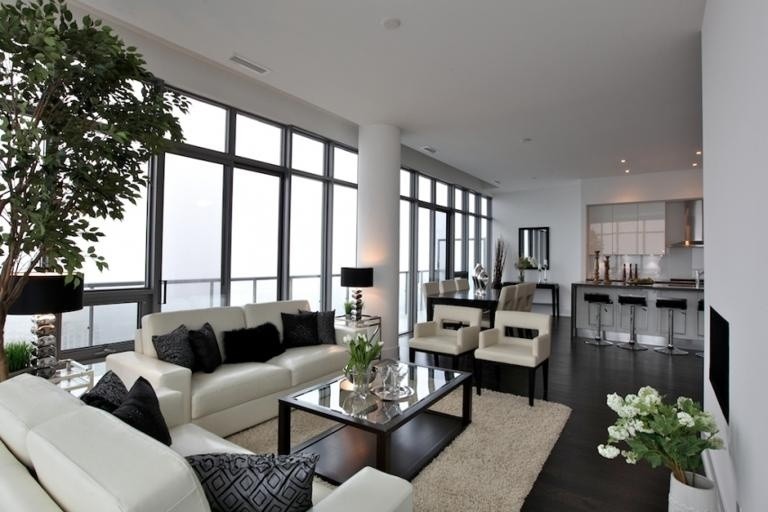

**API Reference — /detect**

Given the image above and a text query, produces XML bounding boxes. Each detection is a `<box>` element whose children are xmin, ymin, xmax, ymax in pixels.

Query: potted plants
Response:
<box><xmin>343</xmin><ymin>301</ymin><xmax>355</xmax><ymax>322</ymax></box>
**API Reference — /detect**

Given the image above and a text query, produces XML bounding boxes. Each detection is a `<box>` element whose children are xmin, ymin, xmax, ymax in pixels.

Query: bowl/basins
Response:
<box><xmin>477</xmin><ymin>278</ymin><xmax>488</xmax><ymax>286</ymax></box>
<box><xmin>472</xmin><ymin>276</ymin><xmax>487</xmax><ymax>288</ymax></box>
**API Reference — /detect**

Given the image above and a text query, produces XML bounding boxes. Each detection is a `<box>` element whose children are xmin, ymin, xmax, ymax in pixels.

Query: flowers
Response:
<box><xmin>340</xmin><ymin>331</ymin><xmax>382</xmax><ymax>371</ymax></box>
<box><xmin>595</xmin><ymin>383</ymin><xmax>729</xmax><ymax>486</ymax></box>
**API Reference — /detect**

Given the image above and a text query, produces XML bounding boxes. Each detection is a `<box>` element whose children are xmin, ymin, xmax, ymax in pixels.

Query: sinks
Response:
<box><xmin>668</xmin><ymin>284</ymin><xmax>703</xmax><ymax>287</ymax></box>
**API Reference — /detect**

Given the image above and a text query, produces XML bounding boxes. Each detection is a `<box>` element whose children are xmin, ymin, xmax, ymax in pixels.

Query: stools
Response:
<box><xmin>694</xmin><ymin>298</ymin><xmax>703</xmax><ymax>359</ymax></box>
<box><xmin>578</xmin><ymin>292</ymin><xmax>613</xmax><ymax>345</ymax></box>
<box><xmin>654</xmin><ymin>297</ymin><xmax>690</xmax><ymax>356</ymax></box>
<box><xmin>617</xmin><ymin>292</ymin><xmax>649</xmax><ymax>350</ymax></box>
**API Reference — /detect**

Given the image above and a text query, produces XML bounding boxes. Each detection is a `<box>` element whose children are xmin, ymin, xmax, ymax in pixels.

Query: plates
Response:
<box><xmin>637</xmin><ymin>282</ymin><xmax>654</xmax><ymax>286</ymax></box>
<box><xmin>370</xmin><ymin>384</ymin><xmax>416</xmax><ymax>401</ymax></box>
<box><xmin>623</xmin><ymin>283</ymin><xmax>638</xmax><ymax>286</ymax></box>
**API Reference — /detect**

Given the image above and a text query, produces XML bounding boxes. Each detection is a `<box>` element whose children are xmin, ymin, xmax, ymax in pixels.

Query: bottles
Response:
<box><xmin>629</xmin><ymin>264</ymin><xmax>632</xmax><ymax>280</ymax></box>
<box><xmin>623</xmin><ymin>264</ymin><xmax>626</xmax><ymax>282</ymax></box>
<box><xmin>592</xmin><ymin>250</ymin><xmax>599</xmax><ymax>282</ymax></box>
<box><xmin>604</xmin><ymin>255</ymin><xmax>615</xmax><ymax>284</ymax></box>
<box><xmin>634</xmin><ymin>263</ymin><xmax>637</xmax><ymax>279</ymax></box>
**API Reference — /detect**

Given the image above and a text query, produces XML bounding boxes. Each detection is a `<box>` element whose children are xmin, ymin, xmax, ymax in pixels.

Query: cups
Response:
<box><xmin>353</xmin><ymin>366</ymin><xmax>369</xmax><ymax>400</ymax></box>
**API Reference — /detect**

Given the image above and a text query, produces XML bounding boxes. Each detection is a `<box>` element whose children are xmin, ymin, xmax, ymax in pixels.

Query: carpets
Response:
<box><xmin>216</xmin><ymin>381</ymin><xmax>575</xmax><ymax>512</ymax></box>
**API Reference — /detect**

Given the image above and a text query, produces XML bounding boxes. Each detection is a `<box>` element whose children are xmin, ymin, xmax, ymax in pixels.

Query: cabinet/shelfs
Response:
<box><xmin>588</xmin><ymin>200</ymin><xmax>665</xmax><ymax>256</ymax></box>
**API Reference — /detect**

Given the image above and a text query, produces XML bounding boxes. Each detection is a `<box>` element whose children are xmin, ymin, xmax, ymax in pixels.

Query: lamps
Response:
<box><xmin>5</xmin><ymin>268</ymin><xmax>84</xmax><ymax>374</ymax></box>
<box><xmin>339</xmin><ymin>265</ymin><xmax>374</xmax><ymax>321</ymax></box>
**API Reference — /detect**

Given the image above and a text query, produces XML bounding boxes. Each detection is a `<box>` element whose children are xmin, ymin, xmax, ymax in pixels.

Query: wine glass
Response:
<box><xmin>376</xmin><ymin>363</ymin><xmax>408</xmax><ymax>395</ymax></box>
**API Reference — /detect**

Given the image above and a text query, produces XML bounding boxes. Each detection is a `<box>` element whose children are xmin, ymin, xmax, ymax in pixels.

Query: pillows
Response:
<box><xmin>111</xmin><ymin>376</ymin><xmax>173</xmax><ymax>447</ymax></box>
<box><xmin>81</xmin><ymin>368</ymin><xmax>128</xmax><ymax>414</ymax></box>
<box><xmin>151</xmin><ymin>310</ymin><xmax>337</xmax><ymax>374</ymax></box>
<box><xmin>189</xmin><ymin>446</ymin><xmax>320</xmax><ymax>512</ymax></box>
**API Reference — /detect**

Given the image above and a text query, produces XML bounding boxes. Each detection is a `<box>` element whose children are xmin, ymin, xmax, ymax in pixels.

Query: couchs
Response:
<box><xmin>104</xmin><ymin>298</ymin><xmax>366</xmax><ymax>441</ymax></box>
<box><xmin>1</xmin><ymin>374</ymin><xmax>412</xmax><ymax>512</ymax></box>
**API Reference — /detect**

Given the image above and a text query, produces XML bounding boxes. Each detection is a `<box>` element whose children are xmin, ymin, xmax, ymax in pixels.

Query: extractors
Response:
<box><xmin>668</xmin><ymin>200</ymin><xmax>704</xmax><ymax>248</ymax></box>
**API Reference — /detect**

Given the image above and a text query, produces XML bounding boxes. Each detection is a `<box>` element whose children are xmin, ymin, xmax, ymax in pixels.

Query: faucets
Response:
<box><xmin>696</xmin><ymin>270</ymin><xmax>704</xmax><ymax>287</ymax></box>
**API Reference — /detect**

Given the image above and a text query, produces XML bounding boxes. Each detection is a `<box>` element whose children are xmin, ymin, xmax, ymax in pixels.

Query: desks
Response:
<box><xmin>503</xmin><ymin>281</ymin><xmax>560</xmax><ymax>320</ymax></box>
<box><xmin>337</xmin><ymin>312</ymin><xmax>383</xmax><ymax>361</ymax></box>
<box><xmin>8</xmin><ymin>356</ymin><xmax>95</xmax><ymax>395</ymax></box>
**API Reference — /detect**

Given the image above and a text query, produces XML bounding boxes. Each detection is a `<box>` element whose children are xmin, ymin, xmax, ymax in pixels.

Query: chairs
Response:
<box><xmin>407</xmin><ymin>304</ymin><xmax>482</xmax><ymax>383</ymax></box>
<box><xmin>473</xmin><ymin>310</ymin><xmax>551</xmax><ymax>408</ymax></box>
<box><xmin>419</xmin><ymin>262</ymin><xmax>537</xmax><ymax>341</ymax></box>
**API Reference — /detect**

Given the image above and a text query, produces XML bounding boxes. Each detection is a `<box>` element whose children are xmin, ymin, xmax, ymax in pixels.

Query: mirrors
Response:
<box><xmin>517</xmin><ymin>226</ymin><xmax>548</xmax><ymax>270</ymax></box>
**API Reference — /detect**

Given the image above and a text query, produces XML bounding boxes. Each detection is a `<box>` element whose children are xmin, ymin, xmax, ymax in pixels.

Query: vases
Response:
<box><xmin>351</xmin><ymin>368</ymin><xmax>368</xmax><ymax>400</ymax></box>
<box><xmin>664</xmin><ymin>470</ymin><xmax>723</xmax><ymax>512</ymax></box>
